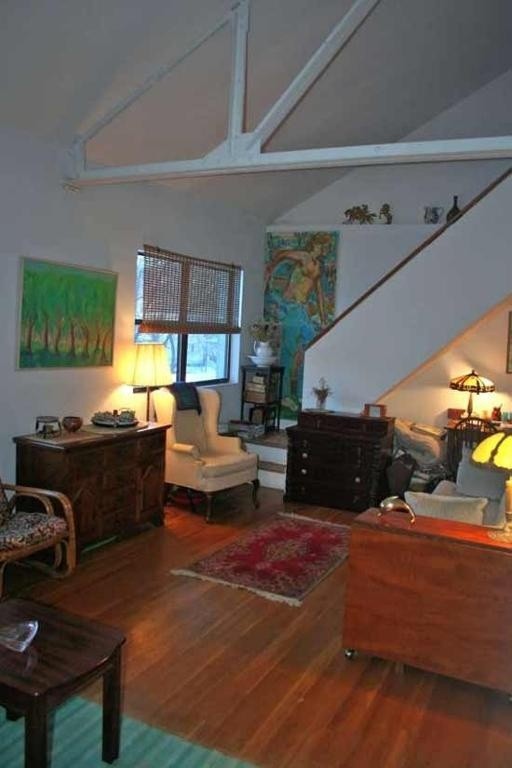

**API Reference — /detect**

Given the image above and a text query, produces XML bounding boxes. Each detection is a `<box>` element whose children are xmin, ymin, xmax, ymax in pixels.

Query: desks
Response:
<box><xmin>444</xmin><ymin>419</ymin><xmax>512</xmax><ymax>474</ymax></box>
<box><xmin>0</xmin><ymin>595</ymin><xmax>127</xmax><ymax>767</ymax></box>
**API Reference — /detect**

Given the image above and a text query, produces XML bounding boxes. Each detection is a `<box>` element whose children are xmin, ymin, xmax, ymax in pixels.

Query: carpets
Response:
<box><xmin>166</xmin><ymin>512</ymin><xmax>350</xmax><ymax>607</ymax></box>
<box><xmin>2</xmin><ymin>695</ymin><xmax>252</xmax><ymax>767</ymax></box>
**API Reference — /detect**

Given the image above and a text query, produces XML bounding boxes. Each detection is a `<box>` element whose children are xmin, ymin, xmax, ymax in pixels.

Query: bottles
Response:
<box><xmin>492</xmin><ymin>408</ymin><xmax>501</xmax><ymax>421</ymax></box>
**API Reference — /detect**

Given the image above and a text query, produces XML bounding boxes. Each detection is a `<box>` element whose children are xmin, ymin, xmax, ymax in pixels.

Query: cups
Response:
<box><xmin>62</xmin><ymin>416</ymin><xmax>82</xmax><ymax>433</ymax></box>
<box><xmin>502</xmin><ymin>412</ymin><xmax>511</xmax><ymax>424</ymax></box>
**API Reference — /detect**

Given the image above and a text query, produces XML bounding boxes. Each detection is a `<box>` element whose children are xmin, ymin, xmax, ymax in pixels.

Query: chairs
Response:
<box><xmin>0</xmin><ymin>479</ymin><xmax>76</xmax><ymax>580</ymax></box>
<box><xmin>152</xmin><ymin>384</ymin><xmax>260</xmax><ymax>522</ymax></box>
<box><xmin>448</xmin><ymin>417</ymin><xmax>498</xmax><ymax>477</ymax></box>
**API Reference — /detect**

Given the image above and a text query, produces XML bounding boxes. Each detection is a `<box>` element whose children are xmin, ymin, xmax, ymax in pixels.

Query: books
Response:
<box><xmin>244</xmin><ymin>375</ymin><xmax>276</xmax><ymax>403</ymax></box>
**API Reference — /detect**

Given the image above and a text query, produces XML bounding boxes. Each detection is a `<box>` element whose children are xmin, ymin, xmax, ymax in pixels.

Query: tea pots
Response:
<box><xmin>422</xmin><ymin>207</ymin><xmax>444</xmax><ymax>224</ymax></box>
<box><xmin>254</xmin><ymin>340</ymin><xmax>273</xmax><ymax>356</ymax></box>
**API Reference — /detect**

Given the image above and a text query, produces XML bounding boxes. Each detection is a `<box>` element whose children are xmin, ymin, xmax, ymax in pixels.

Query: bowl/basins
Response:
<box><xmin>247</xmin><ymin>355</ymin><xmax>278</xmax><ymax>368</ymax></box>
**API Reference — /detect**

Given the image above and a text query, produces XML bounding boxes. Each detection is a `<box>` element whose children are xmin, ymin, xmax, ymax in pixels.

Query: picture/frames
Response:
<box><xmin>16</xmin><ymin>255</ymin><xmax>119</xmax><ymax>371</ymax></box>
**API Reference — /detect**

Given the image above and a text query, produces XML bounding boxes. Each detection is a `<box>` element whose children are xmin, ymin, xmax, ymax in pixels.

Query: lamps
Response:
<box><xmin>126</xmin><ymin>342</ymin><xmax>173</xmax><ymax>422</ymax></box>
<box><xmin>449</xmin><ymin>370</ymin><xmax>494</xmax><ymax>421</ymax></box>
<box><xmin>470</xmin><ymin>429</ymin><xmax>512</xmax><ymax>543</ymax></box>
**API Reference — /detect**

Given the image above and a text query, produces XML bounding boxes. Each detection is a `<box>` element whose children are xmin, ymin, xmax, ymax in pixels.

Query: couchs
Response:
<box><xmin>346</xmin><ymin>445</ymin><xmax>511</xmax><ymax>700</ymax></box>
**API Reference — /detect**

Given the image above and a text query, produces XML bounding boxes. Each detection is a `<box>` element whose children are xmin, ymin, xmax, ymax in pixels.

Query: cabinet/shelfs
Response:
<box><xmin>13</xmin><ymin>420</ymin><xmax>172</xmax><ymax>560</ymax></box>
<box><xmin>282</xmin><ymin>408</ymin><xmax>396</xmax><ymax>514</ymax></box>
<box><xmin>241</xmin><ymin>364</ymin><xmax>284</xmax><ymax>436</ymax></box>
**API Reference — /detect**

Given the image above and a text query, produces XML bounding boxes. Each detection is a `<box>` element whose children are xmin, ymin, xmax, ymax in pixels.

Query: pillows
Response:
<box><xmin>455</xmin><ymin>452</ymin><xmax>512</xmax><ymax>530</ymax></box>
<box><xmin>403</xmin><ymin>491</ymin><xmax>488</xmax><ymax>524</ymax></box>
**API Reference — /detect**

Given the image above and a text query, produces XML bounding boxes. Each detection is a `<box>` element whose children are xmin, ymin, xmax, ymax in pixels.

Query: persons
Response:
<box><xmin>270</xmin><ymin>232</ymin><xmax>325</xmax><ymax>404</ymax></box>
